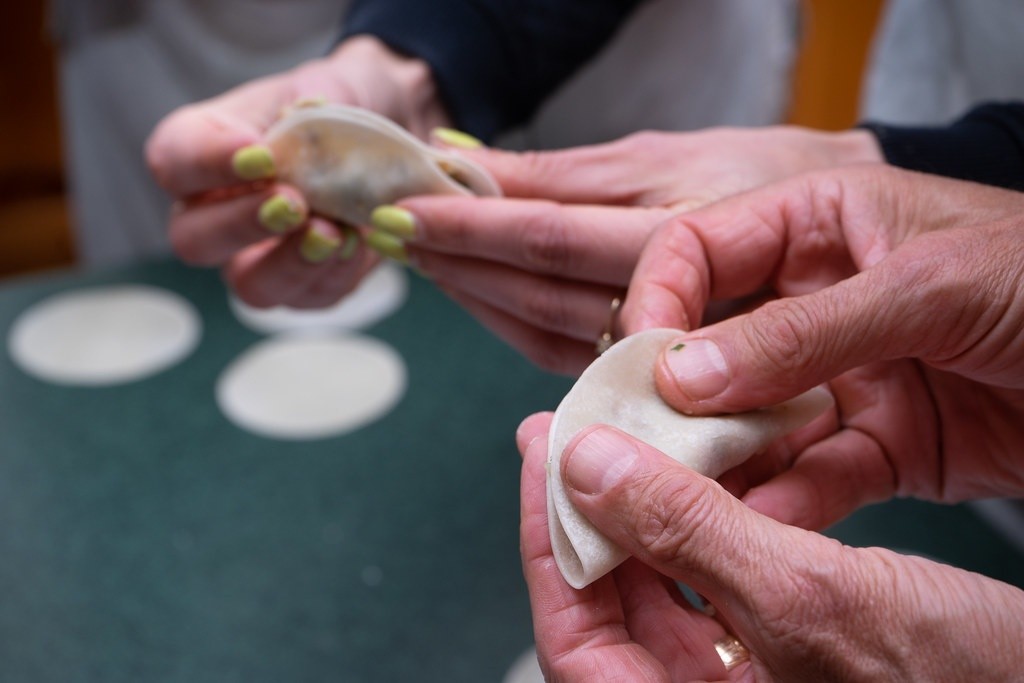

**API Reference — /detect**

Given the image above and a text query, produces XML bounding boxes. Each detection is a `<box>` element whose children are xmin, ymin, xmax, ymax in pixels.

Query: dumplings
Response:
<box><xmin>261</xmin><ymin>102</ymin><xmax>507</xmax><ymax>242</ymax></box>
<box><xmin>545</xmin><ymin>325</ymin><xmax>837</xmax><ymax>589</ymax></box>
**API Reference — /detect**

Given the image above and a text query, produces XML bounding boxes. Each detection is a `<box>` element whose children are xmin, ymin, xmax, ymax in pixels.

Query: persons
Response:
<box><xmin>144</xmin><ymin>1</ymin><xmax>1023</xmax><ymax>379</ymax></box>
<box><xmin>512</xmin><ymin>161</ymin><xmax>1024</xmax><ymax>683</ymax></box>
